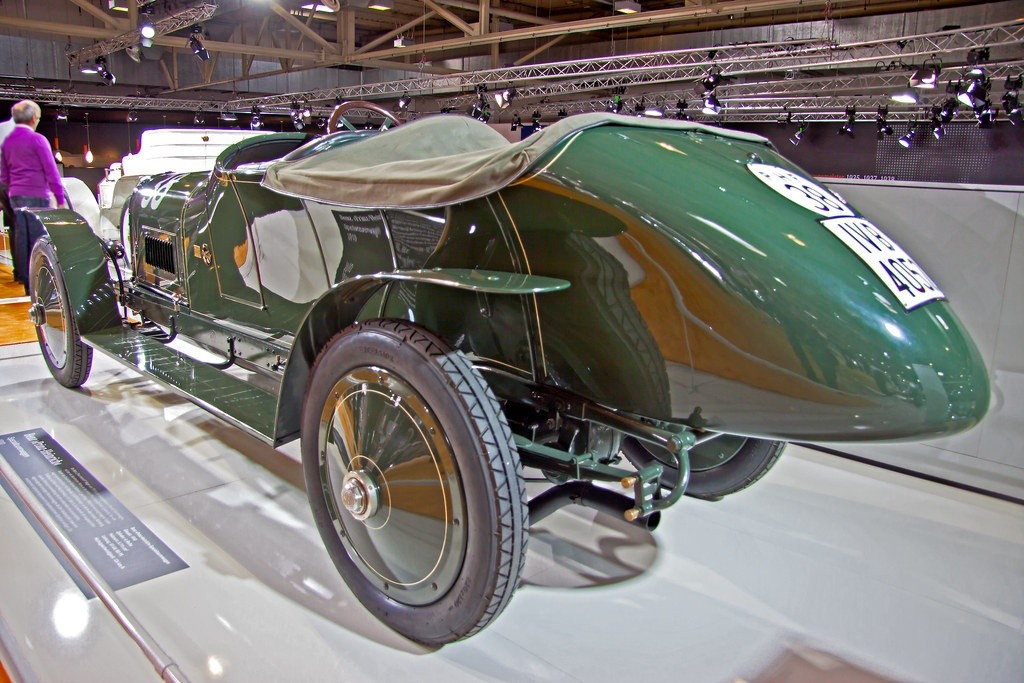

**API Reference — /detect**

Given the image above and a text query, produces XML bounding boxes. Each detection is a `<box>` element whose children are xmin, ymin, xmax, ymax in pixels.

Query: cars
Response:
<box><xmin>18</xmin><ymin>99</ymin><xmax>993</xmax><ymax>647</ymax></box>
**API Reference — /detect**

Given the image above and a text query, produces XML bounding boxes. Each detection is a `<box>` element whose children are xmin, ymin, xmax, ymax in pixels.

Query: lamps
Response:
<box><xmin>251</xmin><ymin>109</ymin><xmax>262</xmax><ymax>127</ymax></box>
<box><xmin>394</xmin><ymin>22</ymin><xmax>416</xmax><ymax>48</ymax></box>
<box><xmin>96</xmin><ymin>57</ymin><xmax>116</xmax><ymax>86</ymax></box>
<box><xmin>472</xmin><ymin>69</ymin><xmax>722</xmax><ymax>131</ymax></box>
<box><xmin>85</xmin><ymin>112</ymin><xmax>94</xmax><ymax>163</ymax></box>
<box><xmin>290</xmin><ymin>96</ymin><xmax>411</xmax><ymax>131</ymax></box>
<box><xmin>54</xmin><ymin>112</ymin><xmax>62</xmax><ymax>162</ymax></box>
<box><xmin>190</xmin><ymin>36</ymin><xmax>210</xmax><ymax>61</ymax></box>
<box><xmin>790</xmin><ymin>123</ymin><xmax>808</xmax><ymax>146</ymax></box>
<box><xmin>128</xmin><ymin>110</ymin><xmax>139</xmax><ymax>121</ymax></box>
<box><xmin>138</xmin><ymin>6</ymin><xmax>156</xmax><ymax>38</ymax></box>
<box><xmin>615</xmin><ymin>0</ymin><xmax>641</xmax><ymax>14</ymax></box>
<box><xmin>837</xmin><ymin>25</ymin><xmax>1024</xmax><ymax>148</ymax></box>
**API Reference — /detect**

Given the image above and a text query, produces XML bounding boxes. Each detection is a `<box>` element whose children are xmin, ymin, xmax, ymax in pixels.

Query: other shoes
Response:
<box><xmin>19</xmin><ymin>277</ymin><xmax>24</xmax><ymax>284</ymax></box>
<box><xmin>14</xmin><ymin>274</ymin><xmax>19</xmax><ymax>281</ymax></box>
<box><xmin>26</xmin><ymin>290</ymin><xmax>30</xmax><ymax>295</ymax></box>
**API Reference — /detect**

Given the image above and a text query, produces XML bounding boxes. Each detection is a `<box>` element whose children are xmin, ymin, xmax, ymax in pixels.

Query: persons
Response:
<box><xmin>0</xmin><ymin>100</ymin><xmax>64</xmax><ymax>296</ymax></box>
